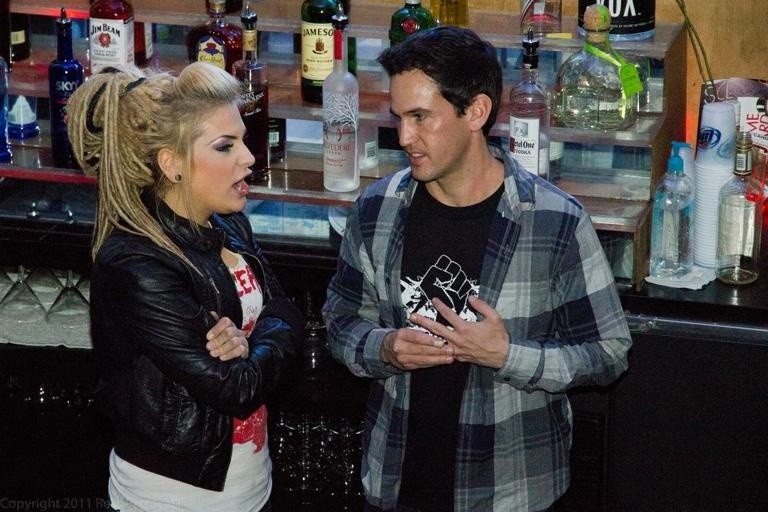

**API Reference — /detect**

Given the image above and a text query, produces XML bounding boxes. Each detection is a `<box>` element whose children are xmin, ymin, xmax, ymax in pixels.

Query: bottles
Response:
<box><xmin>388</xmin><ymin>1</ymin><xmax>440</xmax><ymax>46</ymax></box>
<box><xmin>89</xmin><ymin>1</ymin><xmax>136</xmax><ymax>76</ymax></box>
<box><xmin>228</xmin><ymin>4</ymin><xmax>270</xmax><ymax>183</ymax></box>
<box><xmin>133</xmin><ymin>21</ymin><xmax>156</xmax><ymax>66</ymax></box>
<box><xmin>8</xmin><ymin>12</ymin><xmax>32</xmax><ymax>62</ymax></box>
<box><xmin>519</xmin><ymin>0</ymin><xmax>567</xmax><ymax>31</ymax></box>
<box><xmin>552</xmin><ymin>4</ymin><xmax>644</xmax><ymax>131</ymax></box>
<box><xmin>299</xmin><ymin>0</ymin><xmax>339</xmax><ymax>107</ymax></box>
<box><xmin>575</xmin><ymin>1</ymin><xmax>654</xmax><ymax>42</ymax></box>
<box><xmin>269</xmin><ymin>118</ymin><xmax>288</xmax><ymax>164</ymax></box>
<box><xmin>183</xmin><ymin>1</ymin><xmax>244</xmax><ymax>75</ymax></box>
<box><xmin>46</xmin><ymin>8</ymin><xmax>87</xmax><ymax>169</ymax></box>
<box><xmin>508</xmin><ymin>27</ymin><xmax>552</xmax><ymax>184</ymax></box>
<box><xmin>358</xmin><ymin>122</ymin><xmax>381</xmax><ymax>171</ymax></box>
<box><xmin>320</xmin><ymin>28</ymin><xmax>358</xmax><ymax>193</ymax></box>
<box><xmin>716</xmin><ymin>128</ymin><xmax>767</xmax><ymax>285</ymax></box>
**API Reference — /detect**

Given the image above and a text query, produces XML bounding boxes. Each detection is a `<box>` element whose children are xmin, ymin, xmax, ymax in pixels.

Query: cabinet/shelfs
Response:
<box><xmin>0</xmin><ymin>0</ymin><xmax>689</xmax><ymax>294</ymax></box>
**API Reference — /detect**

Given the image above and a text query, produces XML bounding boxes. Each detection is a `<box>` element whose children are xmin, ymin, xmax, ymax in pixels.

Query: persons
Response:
<box><xmin>318</xmin><ymin>23</ymin><xmax>635</xmax><ymax>511</ymax></box>
<box><xmin>62</xmin><ymin>59</ymin><xmax>311</xmax><ymax>511</ymax></box>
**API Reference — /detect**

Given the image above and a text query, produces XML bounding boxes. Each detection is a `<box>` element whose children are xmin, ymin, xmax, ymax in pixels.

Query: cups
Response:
<box><xmin>692</xmin><ymin>102</ymin><xmax>750</xmax><ymax>274</ymax></box>
